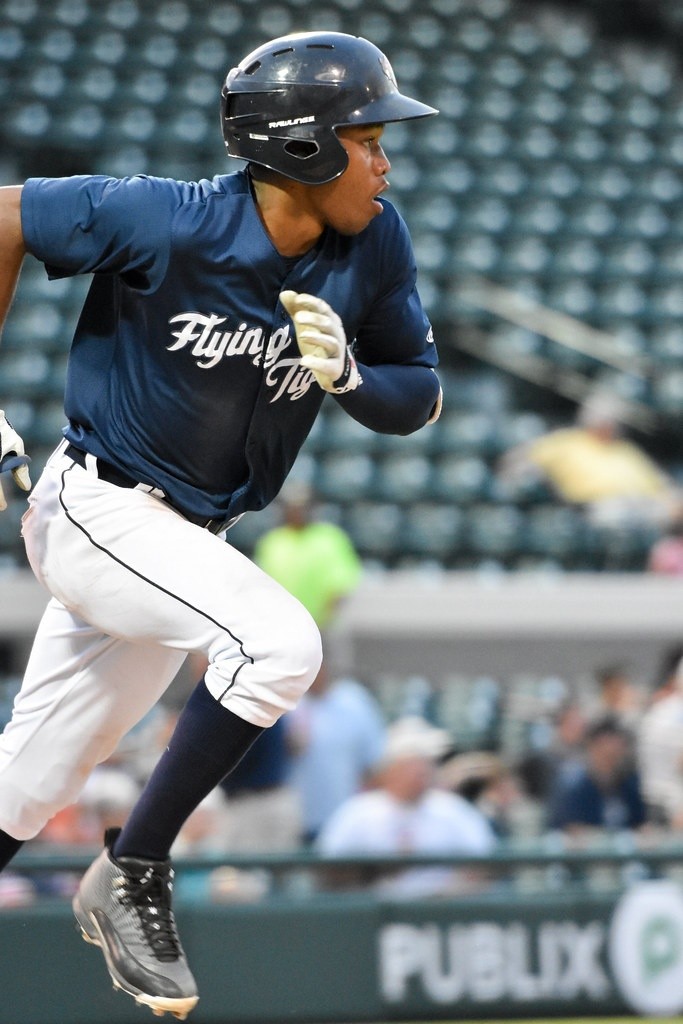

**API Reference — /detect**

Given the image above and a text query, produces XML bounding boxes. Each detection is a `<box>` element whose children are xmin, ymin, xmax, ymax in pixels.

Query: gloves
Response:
<box><xmin>279</xmin><ymin>291</ymin><xmax>360</xmax><ymax>394</ymax></box>
<box><xmin>0</xmin><ymin>410</ymin><xmax>32</xmax><ymax>511</ymax></box>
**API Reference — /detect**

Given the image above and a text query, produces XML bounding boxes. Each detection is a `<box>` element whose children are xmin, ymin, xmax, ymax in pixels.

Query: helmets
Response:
<box><xmin>220</xmin><ymin>30</ymin><xmax>439</xmax><ymax>185</ymax></box>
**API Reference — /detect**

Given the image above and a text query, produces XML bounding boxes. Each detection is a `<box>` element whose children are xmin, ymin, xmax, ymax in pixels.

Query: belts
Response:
<box><xmin>65</xmin><ymin>443</ymin><xmax>224</xmax><ymax>535</ymax></box>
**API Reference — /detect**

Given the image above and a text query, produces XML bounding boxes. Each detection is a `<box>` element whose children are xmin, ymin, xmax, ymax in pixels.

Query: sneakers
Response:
<box><xmin>71</xmin><ymin>826</ymin><xmax>199</xmax><ymax>1020</ymax></box>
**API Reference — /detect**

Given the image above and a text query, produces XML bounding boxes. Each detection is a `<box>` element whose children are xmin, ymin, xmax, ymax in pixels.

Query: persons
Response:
<box><xmin>0</xmin><ymin>33</ymin><xmax>448</xmax><ymax>1017</ymax></box>
<box><xmin>490</xmin><ymin>398</ymin><xmax>682</xmax><ymax>533</ymax></box>
<box><xmin>254</xmin><ymin>478</ymin><xmax>364</xmax><ymax>640</ymax></box>
<box><xmin>1</xmin><ymin>642</ymin><xmax>680</xmax><ymax>902</ymax></box>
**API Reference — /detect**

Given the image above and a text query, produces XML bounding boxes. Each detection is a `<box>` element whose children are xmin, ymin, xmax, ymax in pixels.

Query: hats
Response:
<box><xmin>379</xmin><ymin>718</ymin><xmax>451</xmax><ymax>762</ymax></box>
<box><xmin>442</xmin><ymin>751</ymin><xmax>504</xmax><ymax>787</ymax></box>
<box><xmin>576</xmin><ymin>716</ymin><xmax>638</xmax><ymax>749</ymax></box>
